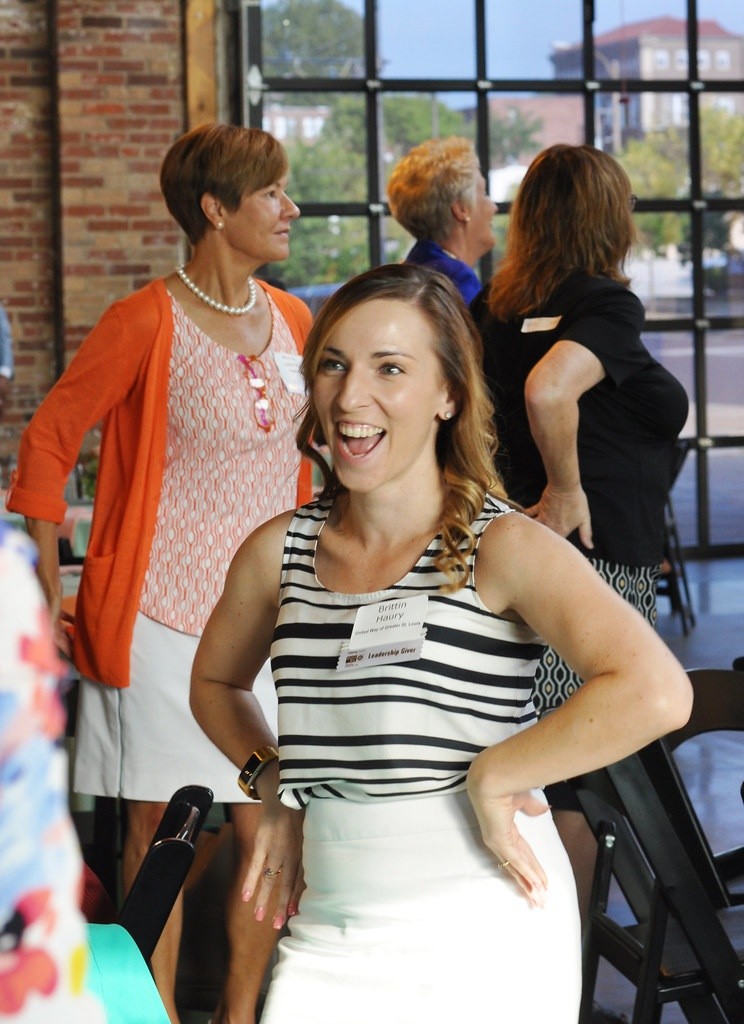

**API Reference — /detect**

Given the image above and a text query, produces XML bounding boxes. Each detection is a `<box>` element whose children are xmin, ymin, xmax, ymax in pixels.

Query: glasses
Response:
<box><xmin>239</xmin><ymin>355</ymin><xmax>274</xmax><ymax>434</ymax></box>
<box><xmin>627</xmin><ymin>192</ymin><xmax>639</xmax><ymax>211</ymax></box>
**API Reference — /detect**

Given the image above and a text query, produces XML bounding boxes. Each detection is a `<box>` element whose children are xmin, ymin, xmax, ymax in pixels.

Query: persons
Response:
<box><xmin>6</xmin><ymin>125</ymin><xmax>319</xmax><ymax>1023</ymax></box>
<box><xmin>190</xmin><ymin>261</ymin><xmax>696</xmax><ymax>1024</ymax></box>
<box><xmin>389</xmin><ymin>135</ymin><xmax>498</xmax><ymax>305</ymax></box>
<box><xmin>468</xmin><ymin>141</ymin><xmax>689</xmax><ymax>885</ymax></box>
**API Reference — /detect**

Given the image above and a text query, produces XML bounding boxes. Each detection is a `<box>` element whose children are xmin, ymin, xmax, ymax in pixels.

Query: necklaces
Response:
<box><xmin>176</xmin><ymin>263</ymin><xmax>259</xmax><ymax>316</ymax></box>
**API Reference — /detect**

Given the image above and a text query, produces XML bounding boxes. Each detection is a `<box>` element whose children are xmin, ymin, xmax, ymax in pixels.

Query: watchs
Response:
<box><xmin>237</xmin><ymin>746</ymin><xmax>281</xmax><ymax>801</ymax></box>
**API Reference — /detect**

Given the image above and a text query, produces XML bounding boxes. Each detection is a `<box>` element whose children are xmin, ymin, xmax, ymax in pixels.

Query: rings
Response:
<box><xmin>263</xmin><ymin>868</ymin><xmax>281</xmax><ymax>877</ymax></box>
<box><xmin>498</xmin><ymin>861</ymin><xmax>511</xmax><ymax>869</ymax></box>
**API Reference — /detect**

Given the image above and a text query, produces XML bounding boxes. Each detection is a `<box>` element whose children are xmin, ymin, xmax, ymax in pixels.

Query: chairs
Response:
<box><xmin>573</xmin><ymin>655</ymin><xmax>744</xmax><ymax>1024</ymax></box>
